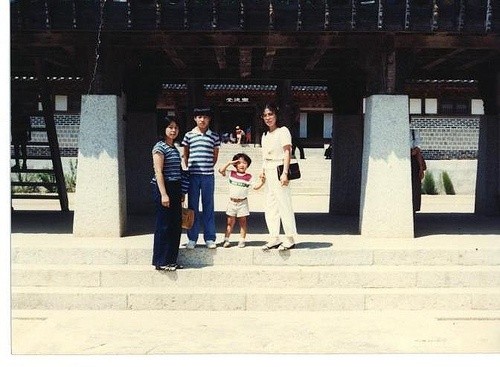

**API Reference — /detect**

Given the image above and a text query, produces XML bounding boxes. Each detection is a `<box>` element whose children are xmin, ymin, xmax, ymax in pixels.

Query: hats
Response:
<box><xmin>236</xmin><ymin>126</ymin><xmax>239</xmax><ymax>129</ymax></box>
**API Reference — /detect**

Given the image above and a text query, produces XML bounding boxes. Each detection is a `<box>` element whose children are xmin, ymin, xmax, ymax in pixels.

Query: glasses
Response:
<box><xmin>261</xmin><ymin>112</ymin><xmax>274</xmax><ymax>118</ymax></box>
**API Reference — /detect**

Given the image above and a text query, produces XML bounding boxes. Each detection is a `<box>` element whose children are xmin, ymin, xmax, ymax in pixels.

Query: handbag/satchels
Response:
<box><xmin>182</xmin><ymin>207</ymin><xmax>195</xmax><ymax>230</ymax></box>
<box><xmin>276</xmin><ymin>158</ymin><xmax>301</xmax><ymax>182</ymax></box>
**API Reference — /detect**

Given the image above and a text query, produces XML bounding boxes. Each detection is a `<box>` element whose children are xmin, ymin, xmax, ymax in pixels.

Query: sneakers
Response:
<box><xmin>205</xmin><ymin>240</ymin><xmax>216</xmax><ymax>249</ymax></box>
<box><xmin>186</xmin><ymin>240</ymin><xmax>197</xmax><ymax>249</ymax></box>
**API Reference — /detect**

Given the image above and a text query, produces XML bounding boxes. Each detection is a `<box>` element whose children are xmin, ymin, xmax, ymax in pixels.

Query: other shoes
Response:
<box><xmin>237</xmin><ymin>240</ymin><xmax>245</xmax><ymax>248</ymax></box>
<box><xmin>155</xmin><ymin>264</ymin><xmax>184</xmax><ymax>271</ymax></box>
<box><xmin>261</xmin><ymin>241</ymin><xmax>282</xmax><ymax>250</ymax></box>
<box><xmin>278</xmin><ymin>242</ymin><xmax>295</xmax><ymax>251</ymax></box>
<box><xmin>223</xmin><ymin>239</ymin><xmax>230</xmax><ymax>247</ymax></box>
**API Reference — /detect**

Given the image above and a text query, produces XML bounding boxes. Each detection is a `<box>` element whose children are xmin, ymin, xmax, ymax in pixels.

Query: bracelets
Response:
<box><xmin>282</xmin><ymin>171</ymin><xmax>289</xmax><ymax>174</ymax></box>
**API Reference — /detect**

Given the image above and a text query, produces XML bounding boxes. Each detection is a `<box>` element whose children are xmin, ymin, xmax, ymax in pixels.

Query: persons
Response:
<box><xmin>292</xmin><ymin>114</ymin><xmax>308</xmax><ymax>160</ymax></box>
<box><xmin>182</xmin><ymin>106</ymin><xmax>220</xmax><ymax>249</ymax></box>
<box><xmin>150</xmin><ymin>116</ymin><xmax>185</xmax><ymax>270</ymax></box>
<box><xmin>324</xmin><ymin>134</ymin><xmax>334</xmax><ymax>160</ymax></box>
<box><xmin>219</xmin><ymin>152</ymin><xmax>265</xmax><ymax>247</ymax></box>
<box><xmin>10</xmin><ymin>105</ymin><xmax>32</xmax><ymax>171</ymax></box>
<box><xmin>260</xmin><ymin>103</ymin><xmax>296</xmax><ymax>251</ymax></box>
<box><xmin>221</xmin><ymin>124</ymin><xmax>251</xmax><ymax>144</ymax></box>
<box><xmin>411</xmin><ymin>147</ymin><xmax>428</xmax><ymax>213</ymax></box>
